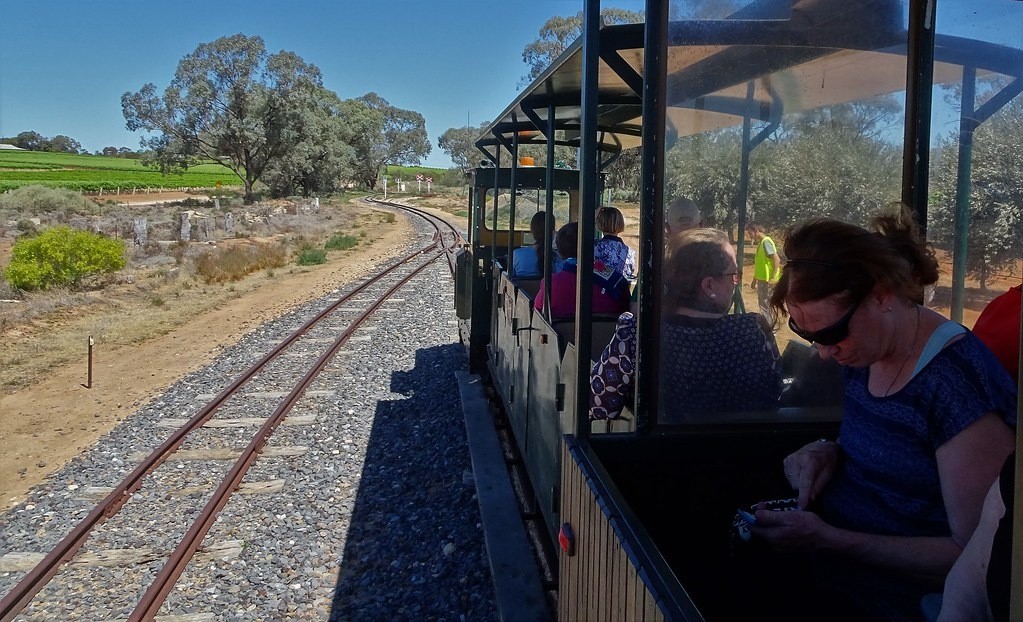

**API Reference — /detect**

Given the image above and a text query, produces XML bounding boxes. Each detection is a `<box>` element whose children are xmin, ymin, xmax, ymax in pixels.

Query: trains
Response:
<box><xmin>451</xmin><ymin>0</ymin><xmax>1023</xmax><ymax>621</ymax></box>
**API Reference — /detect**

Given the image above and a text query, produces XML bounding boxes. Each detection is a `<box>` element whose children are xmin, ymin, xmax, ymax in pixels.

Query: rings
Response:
<box><xmin>788</xmin><ymin>473</ymin><xmax>792</xmax><ymax>476</ymax></box>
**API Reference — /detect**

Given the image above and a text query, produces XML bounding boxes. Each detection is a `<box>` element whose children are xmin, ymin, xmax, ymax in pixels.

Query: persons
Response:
<box><xmin>593</xmin><ymin>206</ymin><xmax>629</xmax><ymax>274</ymax></box>
<box><xmin>588</xmin><ymin>194</ymin><xmax>1023</xmax><ymax>622</ymax></box>
<box><xmin>533</xmin><ymin>221</ymin><xmax>631</xmax><ymax>316</ymax></box>
<box><xmin>513</xmin><ymin>210</ymin><xmax>561</xmax><ymax>279</ymax></box>
<box><xmin>747</xmin><ymin>220</ymin><xmax>782</xmax><ymax>323</ymax></box>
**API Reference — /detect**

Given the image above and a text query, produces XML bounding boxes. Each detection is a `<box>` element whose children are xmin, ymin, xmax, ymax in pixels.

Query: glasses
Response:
<box><xmin>788</xmin><ymin>275</ymin><xmax>877</xmax><ymax>346</ymax></box>
<box><xmin>714</xmin><ymin>268</ymin><xmax>743</xmax><ymax>287</ymax></box>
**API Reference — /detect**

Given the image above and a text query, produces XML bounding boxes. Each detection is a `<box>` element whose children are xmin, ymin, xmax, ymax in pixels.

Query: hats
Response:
<box><xmin>667</xmin><ymin>196</ymin><xmax>701</xmax><ymax>232</ymax></box>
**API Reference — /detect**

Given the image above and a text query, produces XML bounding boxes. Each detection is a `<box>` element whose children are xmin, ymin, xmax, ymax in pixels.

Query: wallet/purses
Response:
<box><xmin>733</xmin><ymin>494</ymin><xmax>799</xmax><ymax>540</ymax></box>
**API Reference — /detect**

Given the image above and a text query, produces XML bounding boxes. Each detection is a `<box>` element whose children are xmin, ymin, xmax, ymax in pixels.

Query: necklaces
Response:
<box><xmin>882</xmin><ymin>302</ymin><xmax>920</xmax><ymax>399</ymax></box>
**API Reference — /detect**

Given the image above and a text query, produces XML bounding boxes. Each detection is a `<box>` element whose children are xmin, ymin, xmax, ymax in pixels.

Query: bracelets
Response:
<box><xmin>820</xmin><ymin>438</ymin><xmax>827</xmax><ymax>442</ymax></box>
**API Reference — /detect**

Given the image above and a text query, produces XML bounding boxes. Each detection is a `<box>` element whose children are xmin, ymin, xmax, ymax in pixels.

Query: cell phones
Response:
<box><xmin>738</xmin><ymin>509</ymin><xmax>759</xmax><ymax>525</ymax></box>
